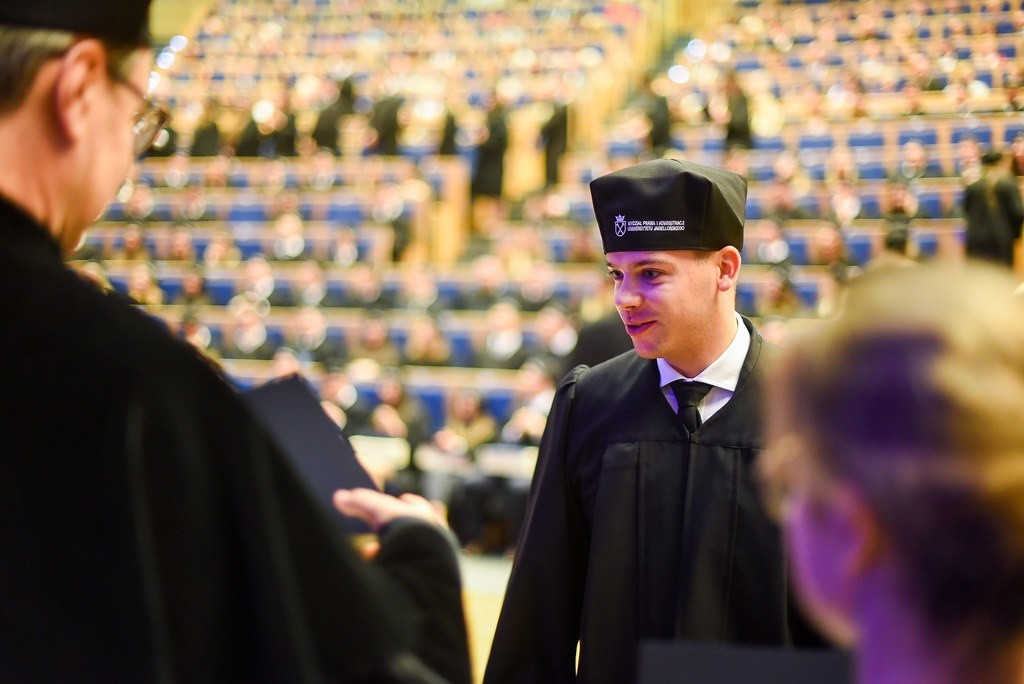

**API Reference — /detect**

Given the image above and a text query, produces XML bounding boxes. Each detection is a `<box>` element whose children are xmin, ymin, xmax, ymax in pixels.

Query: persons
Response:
<box><xmin>761</xmin><ymin>152</ymin><xmax>1024</xmax><ymax>684</ymax></box>
<box><xmin>138</xmin><ymin>67</ymin><xmax>758</xmax><ymax>198</ymax></box>
<box><xmin>483</xmin><ymin>157</ymin><xmax>856</xmax><ymax>684</ymax></box>
<box><xmin>1</xmin><ymin>0</ymin><xmax>474</xmax><ymax>684</ymax></box>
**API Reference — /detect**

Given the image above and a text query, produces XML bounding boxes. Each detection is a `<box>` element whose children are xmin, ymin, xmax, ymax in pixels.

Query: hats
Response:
<box><xmin>589</xmin><ymin>159</ymin><xmax>748</xmax><ymax>255</ymax></box>
<box><xmin>980</xmin><ymin>152</ymin><xmax>1003</xmax><ymax>164</ymax></box>
<box><xmin>0</xmin><ymin>0</ymin><xmax>153</xmax><ymax>51</ymax></box>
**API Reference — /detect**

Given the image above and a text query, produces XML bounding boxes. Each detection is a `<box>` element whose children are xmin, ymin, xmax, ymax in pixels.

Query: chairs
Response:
<box><xmin>69</xmin><ymin>0</ymin><xmax>1024</xmax><ymax>494</ymax></box>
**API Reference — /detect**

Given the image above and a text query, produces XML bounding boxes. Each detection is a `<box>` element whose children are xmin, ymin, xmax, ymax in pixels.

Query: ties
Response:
<box><xmin>668</xmin><ymin>379</ymin><xmax>714</xmax><ymax>434</ymax></box>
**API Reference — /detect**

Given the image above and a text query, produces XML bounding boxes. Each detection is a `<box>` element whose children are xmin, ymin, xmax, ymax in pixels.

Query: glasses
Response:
<box><xmin>42</xmin><ymin>49</ymin><xmax>171</xmax><ymax>162</ymax></box>
